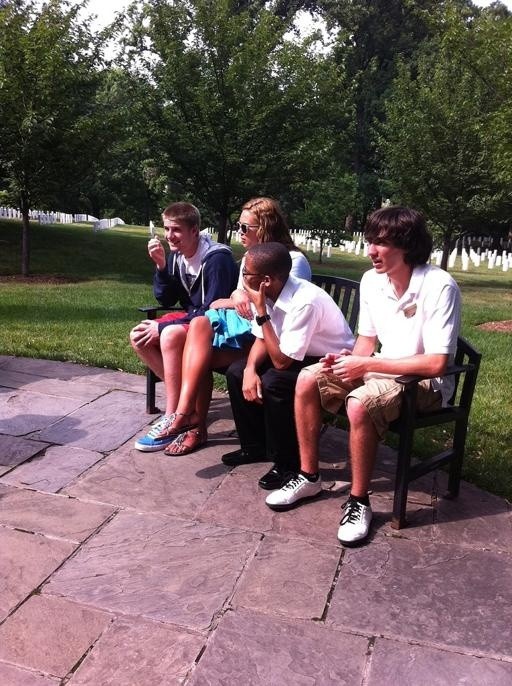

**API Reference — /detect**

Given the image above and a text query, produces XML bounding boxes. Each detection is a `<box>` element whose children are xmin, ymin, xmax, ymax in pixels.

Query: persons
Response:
<box><xmin>129</xmin><ymin>202</ymin><xmax>240</xmax><ymax>451</ymax></box>
<box><xmin>265</xmin><ymin>205</ymin><xmax>463</xmax><ymax>546</ymax></box>
<box><xmin>155</xmin><ymin>196</ymin><xmax>312</xmax><ymax>456</ymax></box>
<box><xmin>221</xmin><ymin>240</ymin><xmax>356</xmax><ymax>490</ymax></box>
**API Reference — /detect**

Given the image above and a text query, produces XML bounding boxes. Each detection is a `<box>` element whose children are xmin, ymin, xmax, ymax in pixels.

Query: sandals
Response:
<box><xmin>164</xmin><ymin>428</ymin><xmax>208</xmax><ymax>456</ymax></box>
<box><xmin>157</xmin><ymin>410</ymin><xmax>201</xmax><ymax>439</ymax></box>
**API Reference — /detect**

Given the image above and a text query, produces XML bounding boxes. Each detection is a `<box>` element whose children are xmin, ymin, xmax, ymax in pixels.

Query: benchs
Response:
<box><xmin>139</xmin><ymin>272</ymin><xmax>483</xmax><ymax>530</ymax></box>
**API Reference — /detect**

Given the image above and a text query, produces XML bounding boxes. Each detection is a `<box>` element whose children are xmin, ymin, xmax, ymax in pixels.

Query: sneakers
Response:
<box><xmin>134</xmin><ymin>412</ymin><xmax>177</xmax><ymax>451</ymax></box>
<box><xmin>265</xmin><ymin>471</ymin><xmax>322</xmax><ymax>508</ymax></box>
<box><xmin>336</xmin><ymin>498</ymin><xmax>372</xmax><ymax>547</ymax></box>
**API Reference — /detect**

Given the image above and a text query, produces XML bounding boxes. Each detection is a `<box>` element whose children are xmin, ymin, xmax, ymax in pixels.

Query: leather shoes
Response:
<box><xmin>221</xmin><ymin>449</ymin><xmax>267</xmax><ymax>465</ymax></box>
<box><xmin>258</xmin><ymin>462</ymin><xmax>294</xmax><ymax>489</ymax></box>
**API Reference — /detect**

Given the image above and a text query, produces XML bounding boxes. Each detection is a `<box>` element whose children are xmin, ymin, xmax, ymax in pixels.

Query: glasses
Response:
<box><xmin>242</xmin><ymin>267</ymin><xmax>259</xmax><ymax>278</ymax></box>
<box><xmin>236</xmin><ymin>221</ymin><xmax>258</xmax><ymax>235</ymax></box>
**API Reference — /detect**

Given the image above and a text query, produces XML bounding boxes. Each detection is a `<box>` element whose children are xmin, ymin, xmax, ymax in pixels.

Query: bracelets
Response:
<box><xmin>255</xmin><ymin>315</ymin><xmax>270</xmax><ymax>326</ymax></box>
